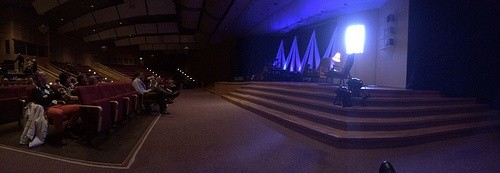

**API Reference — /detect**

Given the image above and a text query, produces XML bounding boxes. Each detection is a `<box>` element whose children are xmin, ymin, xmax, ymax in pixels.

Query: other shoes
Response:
<box><xmin>57</xmin><ymin>139</ymin><xmax>67</xmax><ymax>145</ymax></box>
<box><xmin>65</xmin><ymin>135</ymin><xmax>79</xmax><ymax>139</ymax></box>
<box><xmin>171</xmin><ymin>92</ymin><xmax>180</xmax><ymax>100</ymax></box>
<box><xmin>161</xmin><ymin>111</ymin><xmax>170</xmax><ymax>115</ymax></box>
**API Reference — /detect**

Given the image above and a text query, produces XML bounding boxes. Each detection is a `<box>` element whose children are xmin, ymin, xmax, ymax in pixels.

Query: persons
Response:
<box><xmin>58</xmin><ymin>72</ymin><xmax>78</xmax><ymax>103</ymax></box>
<box><xmin>13</xmin><ymin>53</ymin><xmax>37</xmax><ymax>76</ymax></box>
<box><xmin>56</xmin><ymin>74</ymin><xmax>105</xmax><ymax>86</ymax></box>
<box><xmin>30</xmin><ymin>71</ymin><xmax>79</xmax><ymax>147</ymax></box>
<box><xmin>304</xmin><ymin>64</ymin><xmax>311</xmax><ymax>81</ymax></box>
<box><xmin>133</xmin><ymin>71</ymin><xmax>176</xmax><ymax>115</ymax></box>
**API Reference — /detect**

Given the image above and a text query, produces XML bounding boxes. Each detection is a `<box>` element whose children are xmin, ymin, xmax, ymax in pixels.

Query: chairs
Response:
<box><xmin>0</xmin><ymin>82</ymin><xmax>153</xmax><ymax>139</ymax></box>
